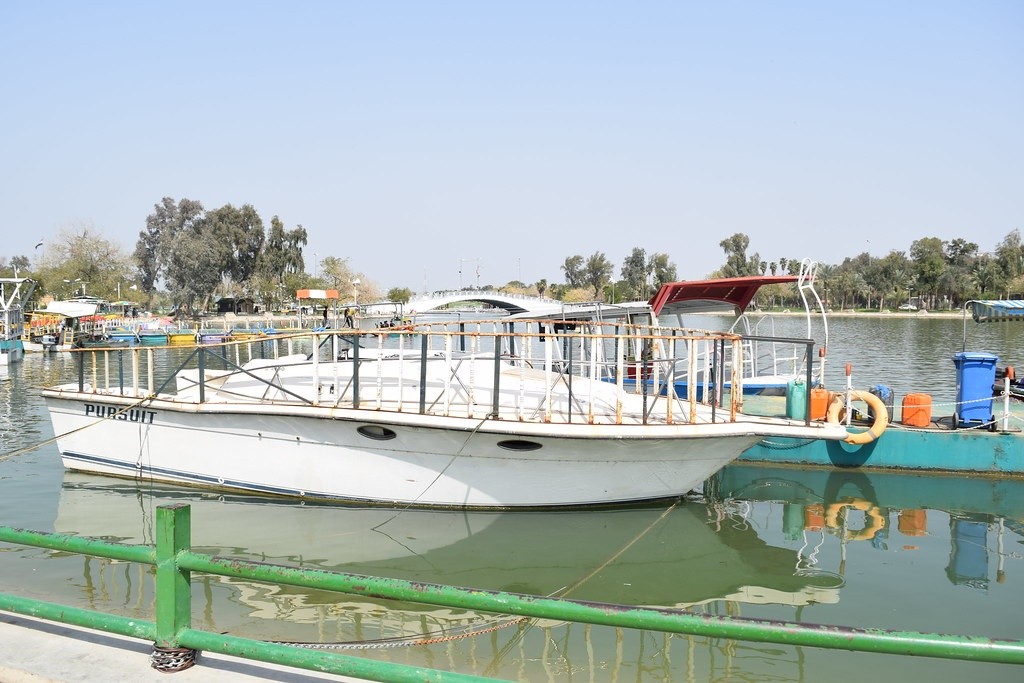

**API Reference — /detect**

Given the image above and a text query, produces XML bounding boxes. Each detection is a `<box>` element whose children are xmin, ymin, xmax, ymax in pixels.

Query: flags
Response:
<box><xmin>35</xmin><ymin>238</ymin><xmax>44</xmax><ymax>249</ymax></box>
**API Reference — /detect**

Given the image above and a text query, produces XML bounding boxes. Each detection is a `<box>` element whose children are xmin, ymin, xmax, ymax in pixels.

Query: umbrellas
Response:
<box><xmin>106</xmin><ymin>300</ymin><xmax>139</xmax><ymax>318</ymax></box>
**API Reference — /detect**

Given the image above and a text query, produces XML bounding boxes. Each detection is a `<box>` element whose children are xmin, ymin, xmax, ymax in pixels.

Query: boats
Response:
<box><xmin>107</xmin><ymin>325</ymin><xmax>285</xmax><ymax>344</ymax></box>
<box><xmin>373</xmin><ymin>327</ymin><xmax>418</xmax><ymax>338</ymax></box>
<box><xmin>579</xmin><ymin>258</ymin><xmax>829</xmax><ymax>403</ymax></box>
<box><xmin>40</xmin><ymin>347</ymin><xmax>849</xmax><ymax>513</ymax></box>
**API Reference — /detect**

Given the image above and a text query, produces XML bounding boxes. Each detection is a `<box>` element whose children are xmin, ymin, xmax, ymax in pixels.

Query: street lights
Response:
<box><xmin>907</xmin><ymin>288</ymin><xmax>914</xmax><ymax>312</ymax></box>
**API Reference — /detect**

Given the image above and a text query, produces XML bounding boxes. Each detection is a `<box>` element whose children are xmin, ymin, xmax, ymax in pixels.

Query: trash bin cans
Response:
<box><xmin>953</xmin><ymin>350</ymin><xmax>1000</xmax><ymax>431</ymax></box>
<box><xmin>944</xmin><ymin>512</ymin><xmax>989</xmax><ymax>591</ymax></box>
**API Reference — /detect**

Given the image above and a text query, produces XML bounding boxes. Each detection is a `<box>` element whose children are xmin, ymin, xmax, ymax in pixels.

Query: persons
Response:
<box><xmin>54</xmin><ymin>333</ymin><xmax>60</xmax><ymax>344</ymax></box>
<box><xmin>379</xmin><ymin>319</ymin><xmax>414</xmax><ymax>336</ymax></box>
<box><xmin>182</xmin><ymin>306</ymin><xmax>188</xmax><ymax>315</ymax></box>
<box><xmin>125</xmin><ymin>305</ymin><xmax>139</xmax><ymax>318</ymax></box>
<box><xmin>345</xmin><ymin>310</ymin><xmax>350</xmax><ymax>327</ymax></box>
<box><xmin>323</xmin><ymin>306</ymin><xmax>328</xmax><ymax>326</ymax></box>
<box><xmin>75</xmin><ymin>338</ymin><xmax>82</xmax><ymax>348</ymax></box>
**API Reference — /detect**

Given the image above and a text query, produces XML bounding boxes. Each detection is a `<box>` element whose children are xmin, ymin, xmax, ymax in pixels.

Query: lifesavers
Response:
<box><xmin>827</xmin><ymin>389</ymin><xmax>888</xmax><ymax>447</ymax></box>
